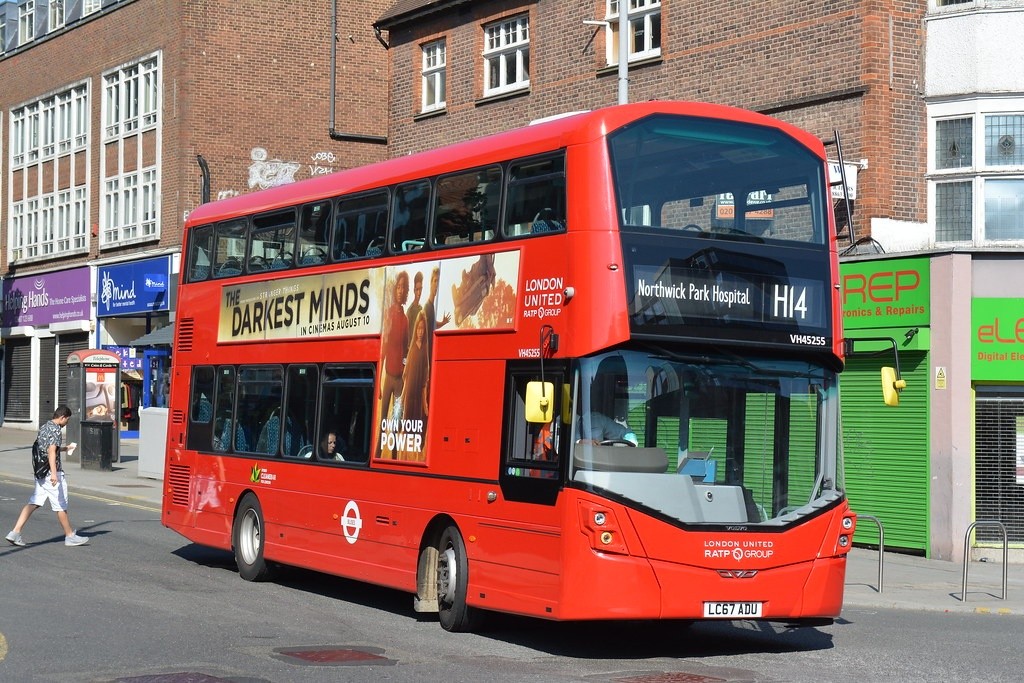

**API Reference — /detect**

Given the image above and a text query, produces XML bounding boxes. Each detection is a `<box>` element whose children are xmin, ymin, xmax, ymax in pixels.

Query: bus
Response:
<box><xmin>161</xmin><ymin>100</ymin><xmax>906</xmax><ymax>633</ymax></box>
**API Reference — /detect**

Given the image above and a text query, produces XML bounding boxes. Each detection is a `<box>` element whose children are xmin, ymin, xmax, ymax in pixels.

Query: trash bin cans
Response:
<box><xmin>80</xmin><ymin>420</ymin><xmax>114</xmax><ymax>471</ymax></box>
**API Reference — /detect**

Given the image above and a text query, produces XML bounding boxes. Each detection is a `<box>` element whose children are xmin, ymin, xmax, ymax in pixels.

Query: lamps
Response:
<box><xmin>904</xmin><ymin>327</ymin><xmax>919</xmax><ymax>339</ymax></box>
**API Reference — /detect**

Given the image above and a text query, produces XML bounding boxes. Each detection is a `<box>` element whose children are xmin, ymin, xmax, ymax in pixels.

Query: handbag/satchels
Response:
<box><xmin>32</xmin><ymin>440</ymin><xmax>51</xmax><ymax>479</ymax></box>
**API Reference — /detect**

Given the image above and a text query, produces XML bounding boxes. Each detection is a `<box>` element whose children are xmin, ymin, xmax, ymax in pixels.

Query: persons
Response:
<box><xmin>375</xmin><ymin>266</ymin><xmax>450</xmax><ymax>460</ymax></box>
<box><xmin>550</xmin><ymin>388</ymin><xmax>639</xmax><ymax>454</ymax></box>
<box><xmin>5</xmin><ymin>405</ymin><xmax>89</xmax><ymax>547</ymax></box>
<box><xmin>304</xmin><ymin>431</ymin><xmax>345</xmax><ymax>461</ymax></box>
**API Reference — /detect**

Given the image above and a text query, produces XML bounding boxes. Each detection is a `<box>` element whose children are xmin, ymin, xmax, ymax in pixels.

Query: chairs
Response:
<box><xmin>533</xmin><ymin>208</ymin><xmax>567</xmax><ymax>233</ymax></box>
<box><xmin>194</xmin><ymin>235</ymin><xmax>445</xmax><ymax>279</ymax></box>
<box><xmin>198</xmin><ymin>391</ymin><xmax>369</xmax><ymax>463</ymax></box>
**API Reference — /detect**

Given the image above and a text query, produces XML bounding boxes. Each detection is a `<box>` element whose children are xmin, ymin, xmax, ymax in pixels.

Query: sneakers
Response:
<box><xmin>5</xmin><ymin>531</ymin><xmax>26</xmax><ymax>546</ymax></box>
<box><xmin>64</xmin><ymin>529</ymin><xmax>90</xmax><ymax>546</ymax></box>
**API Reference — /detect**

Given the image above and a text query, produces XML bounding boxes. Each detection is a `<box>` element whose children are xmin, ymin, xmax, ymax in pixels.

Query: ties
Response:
<box><xmin>579</xmin><ymin>417</ymin><xmax>583</xmax><ymax>439</ymax></box>
